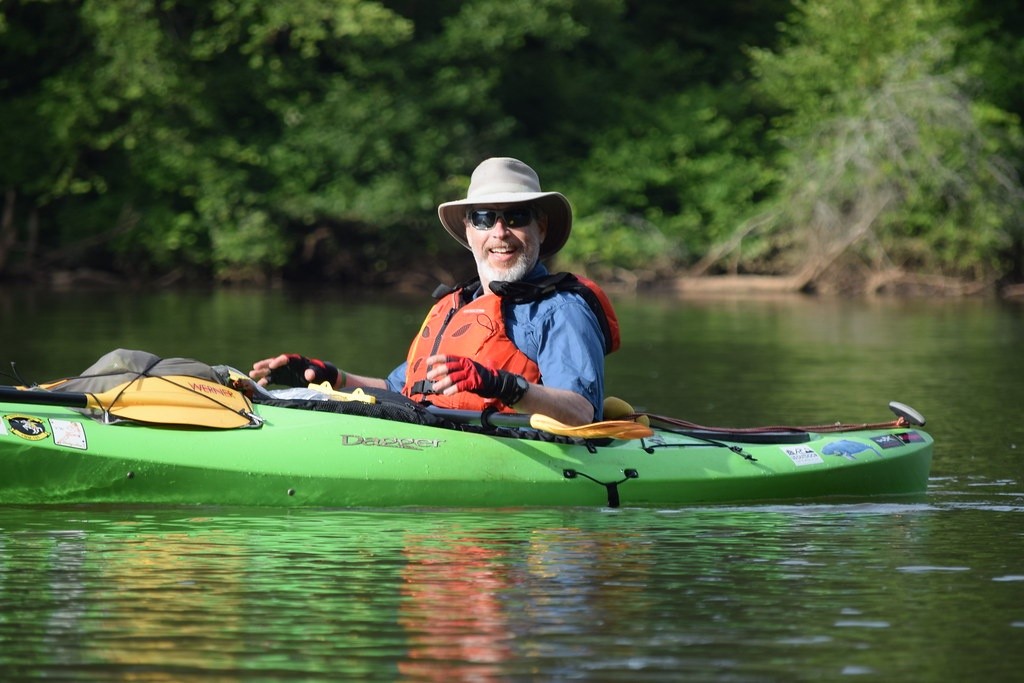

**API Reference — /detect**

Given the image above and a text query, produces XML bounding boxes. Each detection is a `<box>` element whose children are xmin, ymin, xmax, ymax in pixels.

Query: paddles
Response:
<box><xmin>0</xmin><ymin>375</ymin><xmax>255</xmax><ymax>430</ymax></box>
<box><xmin>307</xmin><ymin>380</ymin><xmax>654</xmax><ymax>437</ymax></box>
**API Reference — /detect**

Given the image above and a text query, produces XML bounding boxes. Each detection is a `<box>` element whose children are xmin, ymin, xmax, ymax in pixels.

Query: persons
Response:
<box><xmin>250</xmin><ymin>158</ymin><xmax>620</xmax><ymax>425</ymax></box>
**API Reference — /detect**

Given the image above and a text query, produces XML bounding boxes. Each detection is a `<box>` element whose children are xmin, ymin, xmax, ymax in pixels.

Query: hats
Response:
<box><xmin>438</xmin><ymin>157</ymin><xmax>572</xmax><ymax>260</ymax></box>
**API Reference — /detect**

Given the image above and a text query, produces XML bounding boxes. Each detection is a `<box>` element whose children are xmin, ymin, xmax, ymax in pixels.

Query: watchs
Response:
<box><xmin>510</xmin><ymin>374</ymin><xmax>530</xmax><ymax>406</ymax></box>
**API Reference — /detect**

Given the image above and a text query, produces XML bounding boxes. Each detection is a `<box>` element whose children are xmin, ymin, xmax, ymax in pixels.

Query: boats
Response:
<box><xmin>0</xmin><ymin>387</ymin><xmax>938</xmax><ymax>513</ymax></box>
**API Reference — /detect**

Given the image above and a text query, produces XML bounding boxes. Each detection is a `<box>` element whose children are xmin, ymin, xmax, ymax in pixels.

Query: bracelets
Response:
<box><xmin>337</xmin><ymin>368</ymin><xmax>346</xmax><ymax>389</ymax></box>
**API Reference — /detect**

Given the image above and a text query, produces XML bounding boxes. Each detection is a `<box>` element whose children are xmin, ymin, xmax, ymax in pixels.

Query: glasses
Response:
<box><xmin>465</xmin><ymin>207</ymin><xmax>538</xmax><ymax>230</ymax></box>
